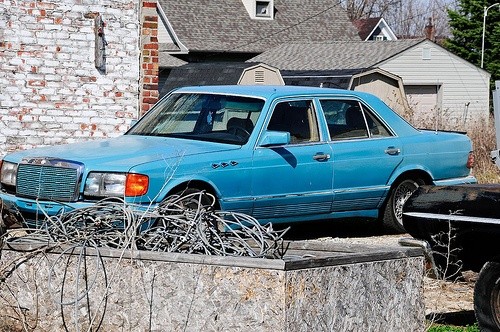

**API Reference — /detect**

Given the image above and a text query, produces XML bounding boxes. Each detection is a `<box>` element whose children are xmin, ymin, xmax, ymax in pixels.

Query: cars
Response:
<box><xmin>0</xmin><ymin>84</ymin><xmax>477</xmax><ymax>239</ymax></box>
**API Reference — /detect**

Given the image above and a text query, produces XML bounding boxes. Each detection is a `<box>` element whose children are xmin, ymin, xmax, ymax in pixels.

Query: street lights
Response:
<box><xmin>480</xmin><ymin>2</ymin><xmax>500</xmax><ymax>70</ymax></box>
